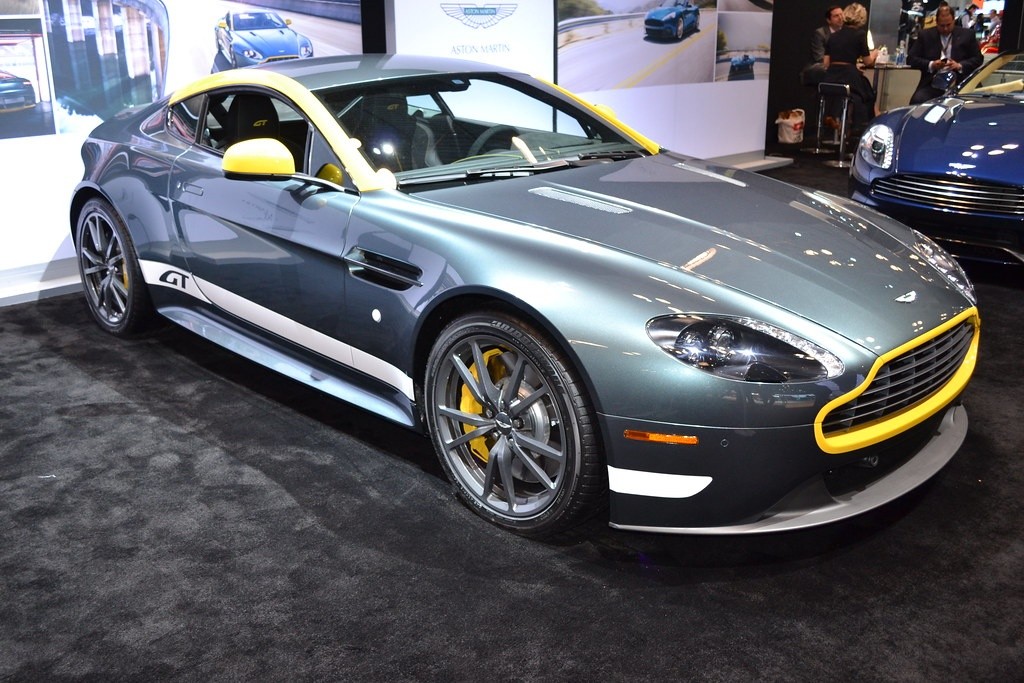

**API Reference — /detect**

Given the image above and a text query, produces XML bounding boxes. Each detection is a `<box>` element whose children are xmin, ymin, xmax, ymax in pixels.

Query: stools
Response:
<box><xmin>800</xmin><ymin>80</ymin><xmax>853</xmax><ymax>168</ymax></box>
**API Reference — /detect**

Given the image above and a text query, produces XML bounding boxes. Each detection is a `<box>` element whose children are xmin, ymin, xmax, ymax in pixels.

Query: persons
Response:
<box><xmin>822</xmin><ymin>3</ymin><xmax>880</xmax><ymax>129</ymax></box>
<box><xmin>801</xmin><ymin>4</ymin><xmax>844</xmax><ymax>87</ymax></box>
<box><xmin>906</xmin><ymin>6</ymin><xmax>989</xmax><ymax>106</ymax></box>
<box><xmin>908</xmin><ymin>1</ymin><xmax>1000</xmax><ymax>51</ymax></box>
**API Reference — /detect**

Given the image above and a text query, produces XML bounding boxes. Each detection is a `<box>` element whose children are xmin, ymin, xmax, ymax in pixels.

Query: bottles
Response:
<box><xmin>881</xmin><ymin>47</ymin><xmax>888</xmax><ymax>56</ymax></box>
<box><xmin>895</xmin><ymin>46</ymin><xmax>906</xmax><ymax>66</ymax></box>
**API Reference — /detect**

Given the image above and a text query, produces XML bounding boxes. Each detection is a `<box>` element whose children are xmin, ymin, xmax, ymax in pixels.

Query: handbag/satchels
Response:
<box><xmin>775</xmin><ymin>109</ymin><xmax>805</xmax><ymax>143</ymax></box>
<box><xmin>932</xmin><ymin>72</ymin><xmax>957</xmax><ymax>93</ymax></box>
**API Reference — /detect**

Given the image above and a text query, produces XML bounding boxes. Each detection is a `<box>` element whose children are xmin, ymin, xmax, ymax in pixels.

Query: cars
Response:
<box><xmin>731</xmin><ymin>55</ymin><xmax>755</xmax><ymax>73</ymax></box>
<box><xmin>644</xmin><ymin>0</ymin><xmax>700</xmax><ymax>40</ymax></box>
<box><xmin>850</xmin><ymin>50</ymin><xmax>1024</xmax><ymax>264</ymax></box>
<box><xmin>0</xmin><ymin>70</ymin><xmax>36</xmax><ymax>113</ymax></box>
<box><xmin>68</xmin><ymin>51</ymin><xmax>982</xmax><ymax>534</ymax></box>
<box><xmin>214</xmin><ymin>8</ymin><xmax>314</xmax><ymax>70</ymax></box>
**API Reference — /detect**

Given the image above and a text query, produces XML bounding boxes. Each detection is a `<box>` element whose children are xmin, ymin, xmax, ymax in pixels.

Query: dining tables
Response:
<box><xmin>860</xmin><ymin>62</ymin><xmax>922</xmax><ymax>113</ymax></box>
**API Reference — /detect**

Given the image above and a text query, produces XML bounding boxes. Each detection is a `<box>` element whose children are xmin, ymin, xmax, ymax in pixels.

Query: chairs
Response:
<box><xmin>224</xmin><ymin>92</ymin><xmax>305</xmax><ymax>173</ymax></box>
<box><xmin>352</xmin><ymin>93</ymin><xmax>418</xmax><ymax>173</ymax></box>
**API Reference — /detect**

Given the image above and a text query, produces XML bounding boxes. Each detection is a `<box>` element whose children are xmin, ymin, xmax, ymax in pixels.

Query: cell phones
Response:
<box><xmin>942</xmin><ymin>58</ymin><xmax>948</xmax><ymax>64</ymax></box>
